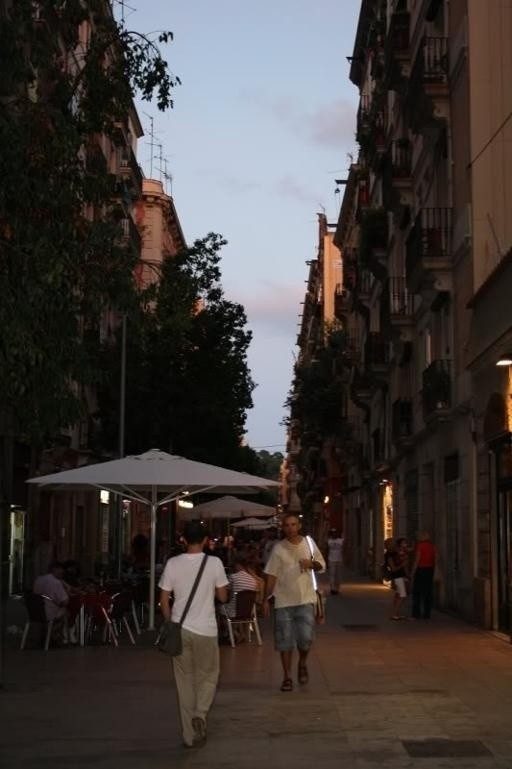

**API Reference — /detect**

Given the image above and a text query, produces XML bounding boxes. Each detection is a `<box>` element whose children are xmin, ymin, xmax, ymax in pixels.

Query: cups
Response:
<box><xmin>299</xmin><ymin>557</ymin><xmax>306</xmax><ymax>574</ymax></box>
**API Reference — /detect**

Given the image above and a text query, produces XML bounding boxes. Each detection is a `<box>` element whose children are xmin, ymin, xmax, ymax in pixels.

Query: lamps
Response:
<box><xmin>494</xmin><ymin>354</ymin><xmax>512</xmax><ymax>368</ymax></box>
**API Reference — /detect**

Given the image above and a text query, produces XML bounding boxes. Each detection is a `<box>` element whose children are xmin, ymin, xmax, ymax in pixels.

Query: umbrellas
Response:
<box><xmin>52</xmin><ymin>484</ymin><xmax>265</xmax><ymax>545</ymax></box>
<box><xmin>179</xmin><ymin>495</ymin><xmax>277</xmax><ymax>546</ymax></box>
<box><xmin>231</xmin><ymin>518</ymin><xmax>276</xmax><ymax>531</ymax></box>
<box><xmin>25</xmin><ymin>449</ymin><xmax>283</xmax><ymax>631</ymax></box>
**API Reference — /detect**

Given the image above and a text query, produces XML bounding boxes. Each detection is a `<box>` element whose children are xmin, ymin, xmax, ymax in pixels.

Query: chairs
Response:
<box><xmin>14</xmin><ymin>575</ymin><xmax>267</xmax><ymax>651</ymax></box>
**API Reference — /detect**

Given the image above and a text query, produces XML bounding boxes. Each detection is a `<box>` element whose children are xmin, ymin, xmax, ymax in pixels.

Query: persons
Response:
<box><xmin>157</xmin><ymin>522</ymin><xmax>230</xmax><ymax>750</ymax></box>
<box><xmin>326</xmin><ymin>527</ymin><xmax>344</xmax><ymax>595</ymax></box>
<box><xmin>31</xmin><ymin>524</ymin><xmax>287</xmax><ymax>646</ymax></box>
<box><xmin>382</xmin><ymin>528</ymin><xmax>439</xmax><ymax>621</ymax></box>
<box><xmin>260</xmin><ymin>515</ymin><xmax>326</xmax><ymax>692</ymax></box>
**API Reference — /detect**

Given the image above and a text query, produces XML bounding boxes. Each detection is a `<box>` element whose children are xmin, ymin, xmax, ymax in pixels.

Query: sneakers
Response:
<box><xmin>391</xmin><ymin>613</ymin><xmax>431</xmax><ymax>622</ymax></box>
<box><xmin>191</xmin><ymin>717</ymin><xmax>208</xmax><ymax>747</ymax></box>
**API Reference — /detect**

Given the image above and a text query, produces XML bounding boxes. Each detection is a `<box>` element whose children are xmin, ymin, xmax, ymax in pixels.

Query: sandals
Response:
<box><xmin>281</xmin><ymin>677</ymin><xmax>292</xmax><ymax>691</ymax></box>
<box><xmin>297</xmin><ymin>662</ymin><xmax>308</xmax><ymax>684</ymax></box>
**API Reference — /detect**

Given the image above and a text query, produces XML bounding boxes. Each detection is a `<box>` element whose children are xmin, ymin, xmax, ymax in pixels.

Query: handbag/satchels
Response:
<box><xmin>153</xmin><ymin>552</ymin><xmax>209</xmax><ymax>656</ymax></box>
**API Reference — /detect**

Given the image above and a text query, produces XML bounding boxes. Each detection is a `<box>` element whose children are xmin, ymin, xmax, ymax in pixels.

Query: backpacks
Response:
<box><xmin>306</xmin><ymin>535</ymin><xmax>327</xmax><ymax>625</ymax></box>
